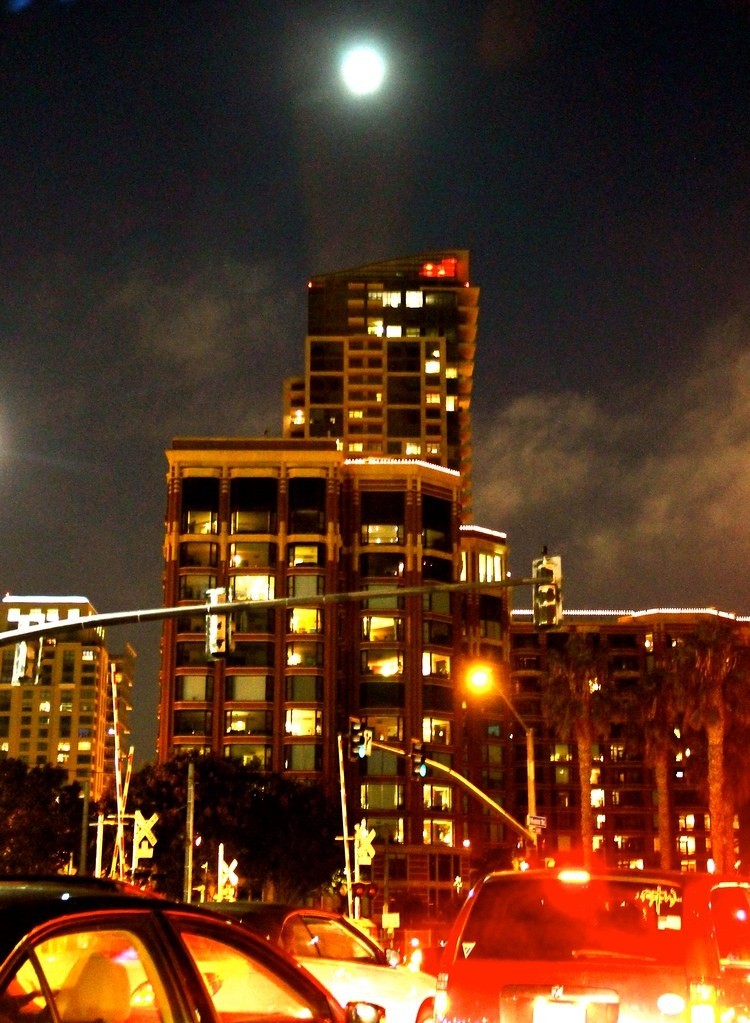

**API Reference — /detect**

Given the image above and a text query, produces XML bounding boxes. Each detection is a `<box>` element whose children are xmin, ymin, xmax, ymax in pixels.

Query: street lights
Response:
<box><xmin>462</xmin><ymin>662</ymin><xmax>538</xmax><ymax>869</ymax></box>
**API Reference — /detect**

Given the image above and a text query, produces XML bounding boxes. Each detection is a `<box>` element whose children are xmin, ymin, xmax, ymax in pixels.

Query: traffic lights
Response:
<box><xmin>193</xmin><ymin>833</ymin><xmax>203</xmax><ymax>849</ymax></box>
<box><xmin>366</xmin><ymin>884</ymin><xmax>378</xmax><ymax>898</ymax></box>
<box><xmin>408</xmin><ymin>737</ymin><xmax>428</xmax><ymax>783</ymax></box>
<box><xmin>347</xmin><ymin>715</ymin><xmax>366</xmax><ymax>763</ymax></box>
<box><xmin>353</xmin><ymin>883</ymin><xmax>366</xmax><ymax>897</ymax></box>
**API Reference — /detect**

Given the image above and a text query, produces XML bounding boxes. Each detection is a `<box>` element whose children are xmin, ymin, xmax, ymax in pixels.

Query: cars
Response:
<box><xmin>0</xmin><ymin>874</ymin><xmax>352</xmax><ymax>1023</ymax></box>
<box><xmin>433</xmin><ymin>871</ymin><xmax>750</xmax><ymax>1023</ymax></box>
<box><xmin>193</xmin><ymin>897</ymin><xmax>445</xmax><ymax>1023</ymax></box>
